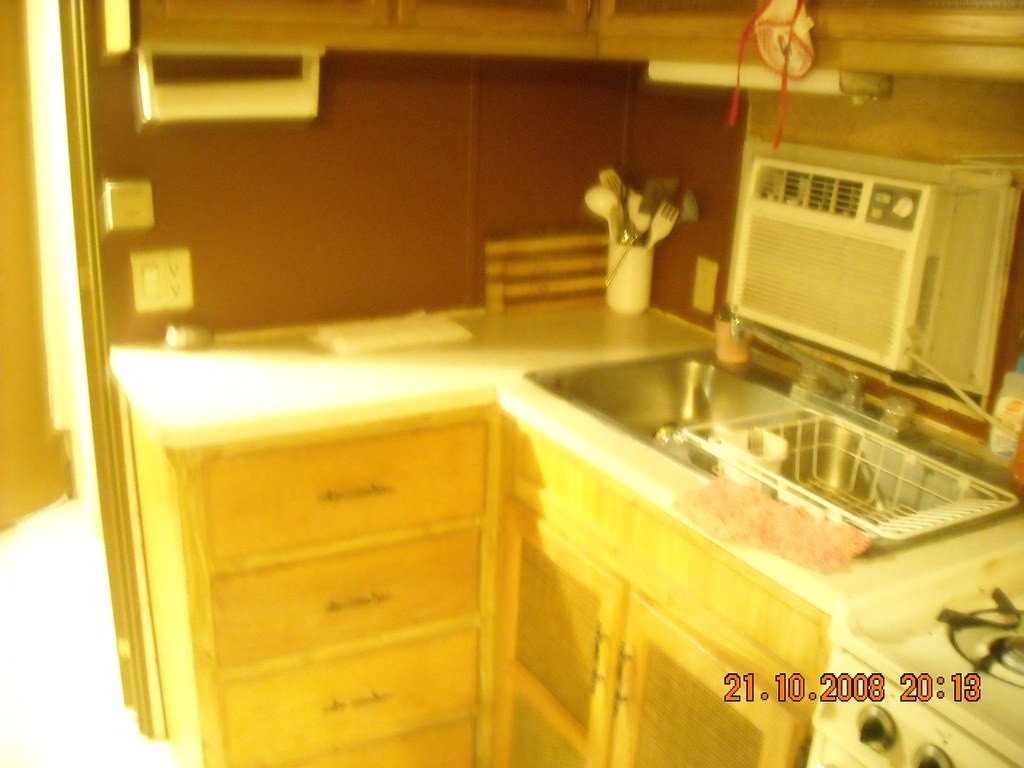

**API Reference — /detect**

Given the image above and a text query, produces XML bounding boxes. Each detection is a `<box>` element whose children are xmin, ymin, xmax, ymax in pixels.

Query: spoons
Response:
<box><xmin>584</xmin><ymin>184</ymin><xmax>619</xmax><ymax>246</ymax></box>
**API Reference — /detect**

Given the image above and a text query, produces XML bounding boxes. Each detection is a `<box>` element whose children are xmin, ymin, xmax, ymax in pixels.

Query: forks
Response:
<box><xmin>645</xmin><ymin>200</ymin><xmax>680</xmax><ymax>247</ymax></box>
<box><xmin>597</xmin><ymin>165</ymin><xmax>627</xmax><ymax>203</ymax></box>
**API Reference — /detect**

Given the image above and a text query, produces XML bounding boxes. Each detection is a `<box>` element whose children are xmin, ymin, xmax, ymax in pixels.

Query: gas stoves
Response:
<box><xmin>802</xmin><ymin>547</ymin><xmax>1024</xmax><ymax>768</ymax></box>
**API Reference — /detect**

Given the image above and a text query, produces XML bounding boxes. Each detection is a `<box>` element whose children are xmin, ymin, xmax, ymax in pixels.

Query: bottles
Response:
<box><xmin>986</xmin><ymin>353</ymin><xmax>1024</xmax><ymax>458</ymax></box>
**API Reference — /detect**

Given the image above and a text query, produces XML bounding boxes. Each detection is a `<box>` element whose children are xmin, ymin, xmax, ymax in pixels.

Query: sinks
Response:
<box><xmin>651</xmin><ymin>404</ymin><xmax>1024</xmax><ymax>556</ymax></box>
<box><xmin>524</xmin><ymin>344</ymin><xmax>811</xmax><ymax>446</ymax></box>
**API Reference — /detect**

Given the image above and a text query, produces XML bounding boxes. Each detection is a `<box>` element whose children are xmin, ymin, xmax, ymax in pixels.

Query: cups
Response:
<box><xmin>714</xmin><ymin>313</ymin><xmax>751</xmax><ymax>366</ymax></box>
<box><xmin>605</xmin><ymin>243</ymin><xmax>654</xmax><ymax>317</ymax></box>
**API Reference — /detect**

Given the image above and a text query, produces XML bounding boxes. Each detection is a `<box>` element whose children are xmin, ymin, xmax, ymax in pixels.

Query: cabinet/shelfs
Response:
<box><xmin>125</xmin><ymin>393</ymin><xmax>834</xmax><ymax>768</ymax></box>
<box><xmin>136</xmin><ymin>1</ymin><xmax>1024</xmax><ymax>77</ymax></box>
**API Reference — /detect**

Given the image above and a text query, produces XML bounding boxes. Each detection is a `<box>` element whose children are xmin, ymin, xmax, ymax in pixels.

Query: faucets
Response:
<box><xmin>727</xmin><ymin>315</ymin><xmax>842</xmax><ymax>389</ymax></box>
